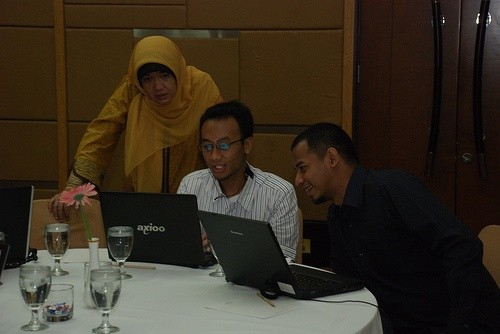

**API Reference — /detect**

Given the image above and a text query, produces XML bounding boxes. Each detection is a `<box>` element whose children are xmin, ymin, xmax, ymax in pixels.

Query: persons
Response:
<box><xmin>176</xmin><ymin>99</ymin><xmax>300</xmax><ymax>265</ymax></box>
<box><xmin>48</xmin><ymin>35</ymin><xmax>224</xmax><ymax>221</ymax></box>
<box><xmin>290</xmin><ymin>122</ymin><xmax>500</xmax><ymax>334</ymax></box>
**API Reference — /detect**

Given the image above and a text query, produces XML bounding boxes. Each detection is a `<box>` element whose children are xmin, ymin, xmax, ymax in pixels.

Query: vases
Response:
<box><xmin>83</xmin><ymin>238</ymin><xmax>101</xmax><ymax>308</ymax></box>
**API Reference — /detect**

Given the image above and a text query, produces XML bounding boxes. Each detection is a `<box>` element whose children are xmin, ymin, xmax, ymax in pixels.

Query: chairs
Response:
<box><xmin>28</xmin><ymin>198</ymin><xmax>107</xmax><ymax>250</ymax></box>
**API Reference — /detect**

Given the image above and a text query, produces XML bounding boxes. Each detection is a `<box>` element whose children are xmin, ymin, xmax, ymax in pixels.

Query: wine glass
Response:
<box><xmin>108</xmin><ymin>227</ymin><xmax>134</xmax><ymax>281</ymax></box>
<box><xmin>44</xmin><ymin>222</ymin><xmax>71</xmax><ymax>276</ymax></box>
<box><xmin>90</xmin><ymin>268</ymin><xmax>121</xmax><ymax>334</ymax></box>
<box><xmin>19</xmin><ymin>265</ymin><xmax>51</xmax><ymax>331</ymax></box>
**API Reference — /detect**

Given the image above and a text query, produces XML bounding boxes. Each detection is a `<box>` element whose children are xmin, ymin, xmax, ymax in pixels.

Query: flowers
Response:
<box><xmin>59</xmin><ymin>182</ymin><xmax>99</xmax><ymax>241</ymax></box>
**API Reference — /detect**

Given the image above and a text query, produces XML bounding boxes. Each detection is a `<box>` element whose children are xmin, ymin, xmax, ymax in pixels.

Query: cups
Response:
<box><xmin>42</xmin><ymin>284</ymin><xmax>75</xmax><ymax>322</ymax></box>
<box><xmin>84</xmin><ymin>262</ymin><xmax>111</xmax><ymax>286</ymax></box>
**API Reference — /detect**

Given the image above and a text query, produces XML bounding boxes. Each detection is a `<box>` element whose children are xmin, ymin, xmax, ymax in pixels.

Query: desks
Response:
<box><xmin>0</xmin><ymin>246</ymin><xmax>382</xmax><ymax>334</ymax></box>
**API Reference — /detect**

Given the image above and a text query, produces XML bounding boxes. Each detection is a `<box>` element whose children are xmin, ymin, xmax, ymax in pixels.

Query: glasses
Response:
<box><xmin>199</xmin><ymin>138</ymin><xmax>241</xmax><ymax>152</ymax></box>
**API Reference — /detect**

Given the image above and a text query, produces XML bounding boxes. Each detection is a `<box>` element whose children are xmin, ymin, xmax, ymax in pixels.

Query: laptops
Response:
<box><xmin>0</xmin><ymin>185</ymin><xmax>34</xmax><ymax>277</ymax></box>
<box><xmin>197</xmin><ymin>209</ymin><xmax>366</xmax><ymax>300</ymax></box>
<box><xmin>99</xmin><ymin>191</ymin><xmax>217</xmax><ymax>269</ymax></box>
